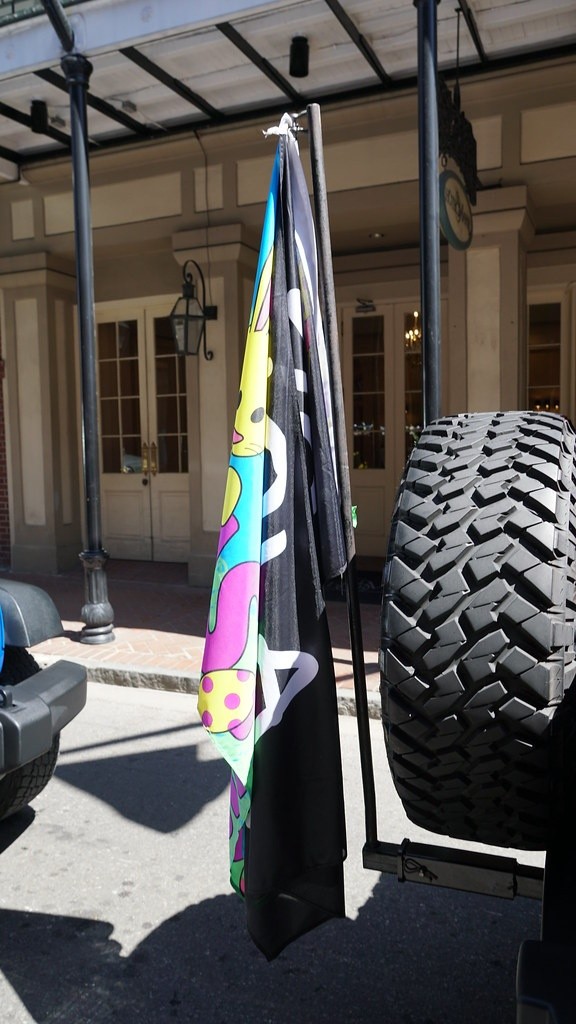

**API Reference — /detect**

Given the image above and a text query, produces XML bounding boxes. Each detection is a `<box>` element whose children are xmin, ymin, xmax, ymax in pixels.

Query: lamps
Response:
<box><xmin>289</xmin><ymin>36</ymin><xmax>309</xmax><ymax>78</ymax></box>
<box><xmin>405</xmin><ymin>312</ymin><xmax>422</xmax><ymax>368</ymax></box>
<box><xmin>30</xmin><ymin>100</ymin><xmax>49</xmax><ymax>134</ymax></box>
<box><xmin>168</xmin><ymin>259</ymin><xmax>218</xmax><ymax>362</ymax></box>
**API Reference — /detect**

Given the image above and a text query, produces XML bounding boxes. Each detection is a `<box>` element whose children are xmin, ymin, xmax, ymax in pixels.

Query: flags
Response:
<box><xmin>196</xmin><ymin>134</ymin><xmax>348</xmax><ymax>962</ymax></box>
